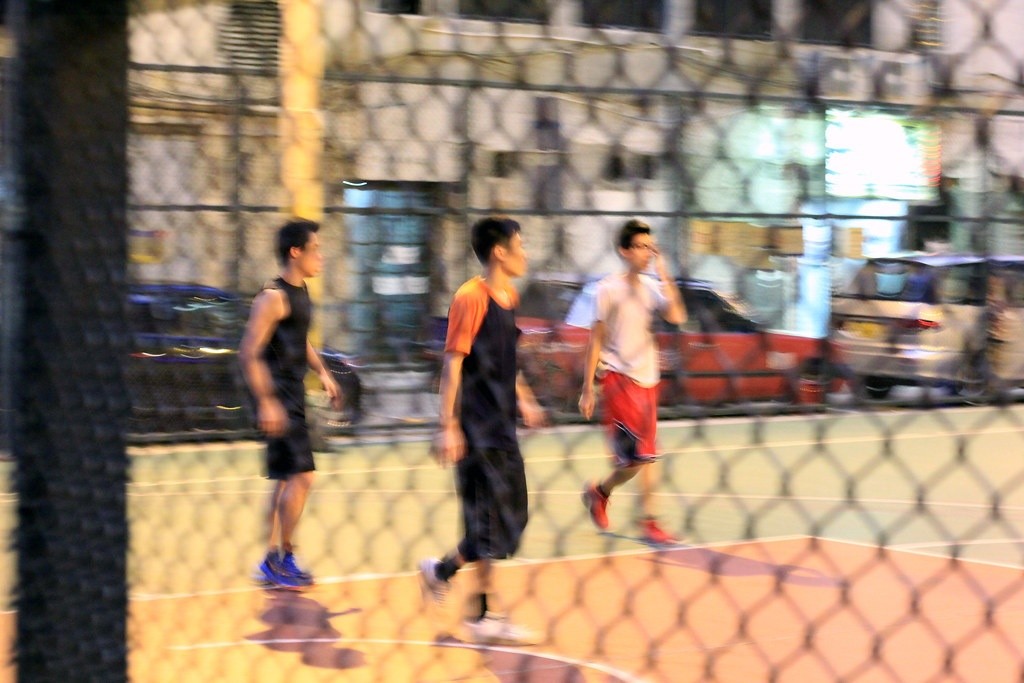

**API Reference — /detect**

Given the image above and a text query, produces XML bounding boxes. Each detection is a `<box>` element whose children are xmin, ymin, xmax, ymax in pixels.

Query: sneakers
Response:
<box><xmin>259</xmin><ymin>552</ymin><xmax>303</xmax><ymax>587</ymax></box>
<box><xmin>417</xmin><ymin>558</ymin><xmax>450</xmax><ymax>611</ymax></box>
<box><xmin>282</xmin><ymin>553</ymin><xmax>312</xmax><ymax>583</ymax></box>
<box><xmin>453</xmin><ymin>612</ymin><xmax>543</xmax><ymax>645</ymax></box>
<box><xmin>582</xmin><ymin>481</ymin><xmax>612</xmax><ymax>531</ymax></box>
<box><xmin>635</xmin><ymin>516</ymin><xmax>684</xmax><ymax>545</ymax></box>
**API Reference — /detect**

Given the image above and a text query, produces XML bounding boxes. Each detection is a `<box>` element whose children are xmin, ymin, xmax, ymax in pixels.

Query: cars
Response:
<box><xmin>829</xmin><ymin>252</ymin><xmax>1024</xmax><ymax>409</ymax></box>
<box><xmin>122</xmin><ymin>277</ymin><xmax>362</xmax><ymax>441</ymax></box>
<box><xmin>418</xmin><ymin>276</ymin><xmax>842</xmax><ymax>406</ymax></box>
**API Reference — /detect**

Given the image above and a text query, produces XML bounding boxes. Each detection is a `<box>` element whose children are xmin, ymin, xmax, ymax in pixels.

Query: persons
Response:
<box><xmin>238</xmin><ymin>219</ymin><xmax>342</xmax><ymax>593</ymax></box>
<box><xmin>579</xmin><ymin>220</ymin><xmax>688</xmax><ymax>543</ymax></box>
<box><xmin>414</xmin><ymin>216</ymin><xmax>545</xmax><ymax>641</ymax></box>
